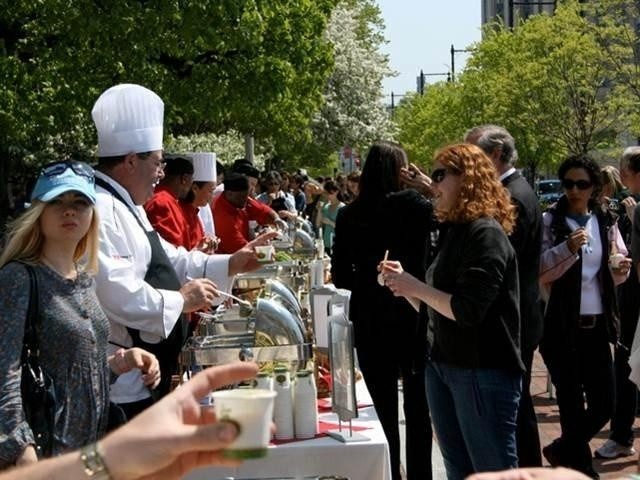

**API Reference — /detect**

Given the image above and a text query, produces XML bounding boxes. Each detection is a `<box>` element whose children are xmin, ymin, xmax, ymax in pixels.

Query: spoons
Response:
<box><xmin>186</xmin><ymin>275</ymin><xmax>254</xmax><ymax>318</ymax></box>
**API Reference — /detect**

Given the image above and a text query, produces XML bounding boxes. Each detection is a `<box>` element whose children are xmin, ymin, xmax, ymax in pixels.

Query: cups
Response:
<box><xmin>254</xmin><ymin>246</ymin><xmax>273</xmax><ymax>262</ymax></box>
<box><xmin>210</xmin><ymin>388</ymin><xmax>278</xmax><ymax>460</ymax></box>
<box><xmin>610</xmin><ymin>253</ymin><xmax>626</xmax><ymax>270</ymax></box>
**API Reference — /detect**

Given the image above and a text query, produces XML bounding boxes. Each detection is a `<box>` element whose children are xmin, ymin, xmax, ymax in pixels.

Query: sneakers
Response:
<box><xmin>594</xmin><ymin>436</ymin><xmax>636</xmax><ymax>460</ymax></box>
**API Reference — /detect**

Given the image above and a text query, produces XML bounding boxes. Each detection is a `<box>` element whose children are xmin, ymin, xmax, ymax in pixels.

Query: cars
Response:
<box><xmin>536</xmin><ymin>179</ymin><xmax>566</xmax><ymax>203</ymax></box>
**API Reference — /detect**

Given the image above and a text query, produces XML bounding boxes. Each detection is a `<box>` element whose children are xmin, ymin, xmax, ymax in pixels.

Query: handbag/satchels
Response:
<box><xmin>6</xmin><ymin>258</ymin><xmax>58</xmax><ymax>461</ymax></box>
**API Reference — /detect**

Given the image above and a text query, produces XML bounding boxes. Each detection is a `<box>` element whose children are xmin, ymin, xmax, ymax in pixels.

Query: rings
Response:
<box><xmin>411</xmin><ymin>171</ymin><xmax>417</xmax><ymax>178</ymax></box>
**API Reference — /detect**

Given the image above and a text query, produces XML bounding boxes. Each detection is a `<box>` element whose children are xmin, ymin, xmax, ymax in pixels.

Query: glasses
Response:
<box><xmin>151</xmin><ymin>159</ymin><xmax>168</xmax><ymax>170</ymax></box>
<box><xmin>430</xmin><ymin>168</ymin><xmax>461</xmax><ymax>183</ymax></box>
<box><xmin>42</xmin><ymin>161</ymin><xmax>96</xmax><ymax>179</ymax></box>
<box><xmin>561</xmin><ymin>179</ymin><xmax>593</xmax><ymax>190</ymax></box>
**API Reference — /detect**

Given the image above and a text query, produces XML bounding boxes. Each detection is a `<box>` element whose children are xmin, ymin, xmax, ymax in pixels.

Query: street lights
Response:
<box><xmin>451</xmin><ymin>43</ymin><xmax>479</xmax><ymax>82</ymax></box>
<box><xmin>421</xmin><ymin>70</ymin><xmax>451</xmax><ymax>97</ymax></box>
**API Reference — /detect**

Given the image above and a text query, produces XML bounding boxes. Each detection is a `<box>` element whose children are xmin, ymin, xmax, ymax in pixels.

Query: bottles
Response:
<box><xmin>274</xmin><ymin>370</ymin><xmax>293</xmax><ymax>441</ymax></box>
<box><xmin>295</xmin><ymin>374</ymin><xmax>317</xmax><ymax>439</ymax></box>
<box><xmin>256</xmin><ymin>374</ymin><xmax>270</xmax><ymax>392</ymax></box>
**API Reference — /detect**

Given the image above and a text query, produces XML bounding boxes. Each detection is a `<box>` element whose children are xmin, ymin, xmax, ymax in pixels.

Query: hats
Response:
<box><xmin>31</xmin><ymin>160</ymin><xmax>97</xmax><ymax>204</ymax></box>
<box><xmin>192</xmin><ymin>152</ymin><xmax>217</xmax><ymax>183</ymax></box>
<box><xmin>91</xmin><ymin>83</ymin><xmax>164</xmax><ymax>158</ymax></box>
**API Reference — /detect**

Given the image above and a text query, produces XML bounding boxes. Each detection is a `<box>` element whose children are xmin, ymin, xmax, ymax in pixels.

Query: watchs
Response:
<box><xmin>79</xmin><ymin>442</ymin><xmax>111</xmax><ymax>480</ymax></box>
<box><xmin>114</xmin><ymin>347</ymin><xmax>130</xmax><ymax>373</ymax></box>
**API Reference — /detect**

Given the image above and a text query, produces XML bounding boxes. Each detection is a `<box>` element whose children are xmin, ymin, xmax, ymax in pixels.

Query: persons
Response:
<box><xmin>77</xmin><ymin>84</ymin><xmax>279</xmax><ymax>431</ymax></box>
<box><xmin>198</xmin><ymin>159</ymin><xmax>225</xmax><ymax>238</ymax></box>
<box><xmin>0</xmin><ymin>159</ymin><xmax>161</xmax><ymax>470</ymax></box>
<box><xmin>594</xmin><ymin>145</ymin><xmax>640</xmax><ymax>461</ymax></box>
<box><xmin>316</xmin><ymin>181</ymin><xmax>347</xmax><ymax>255</ymax></box>
<box><xmin>539</xmin><ymin>155</ymin><xmax>634</xmax><ymax>480</ymax></box>
<box><xmin>0</xmin><ymin>361</ymin><xmax>278</xmax><ymax>480</ymax></box>
<box><xmin>464</xmin><ymin>124</ymin><xmax>545</xmax><ymax>467</ymax></box>
<box><xmin>336</xmin><ymin>174</ymin><xmax>352</xmax><ymax>203</ymax></box>
<box><xmin>255</xmin><ymin>171</ymin><xmax>298</xmax><ymax>223</ymax></box>
<box><xmin>210</xmin><ymin>174</ymin><xmax>289</xmax><ymax>254</ymax></box>
<box><xmin>280</xmin><ymin>170</ymin><xmax>296</xmax><ymax>209</ymax></box>
<box><xmin>292</xmin><ymin>173</ymin><xmax>306</xmax><ymax>216</ymax></box>
<box><xmin>376</xmin><ymin>143</ymin><xmax>526</xmax><ymax>480</ymax></box>
<box><xmin>346</xmin><ymin>169</ymin><xmax>362</xmax><ymax>204</ymax></box>
<box><xmin>601</xmin><ymin>165</ymin><xmax>633</xmax><ymax>200</ymax></box>
<box><xmin>144</xmin><ymin>152</ymin><xmax>219</xmax><ymax>322</ymax></box>
<box><xmin>331</xmin><ymin>140</ymin><xmax>438</xmax><ymax>480</ymax></box>
<box><xmin>234</xmin><ymin>162</ymin><xmax>261</xmax><ymax>199</ymax></box>
<box><xmin>178</xmin><ymin>151</ymin><xmax>218</xmax><ymax>249</ymax></box>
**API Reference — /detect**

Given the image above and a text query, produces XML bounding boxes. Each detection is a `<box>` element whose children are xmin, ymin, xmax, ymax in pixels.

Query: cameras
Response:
<box><xmin>607</xmin><ymin>198</ymin><xmax>627</xmax><ymax>214</ymax></box>
